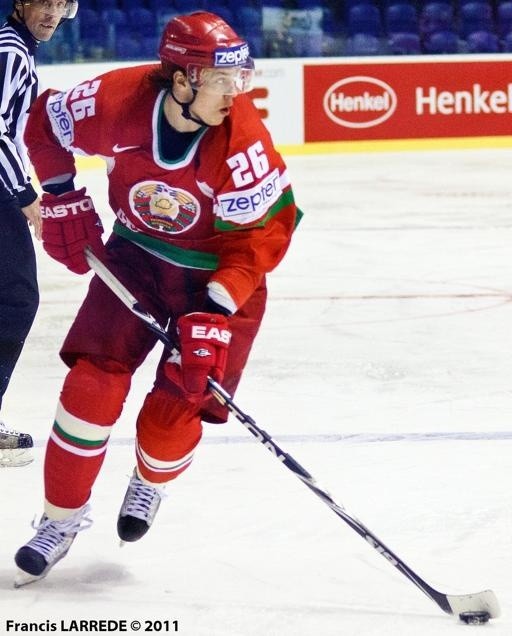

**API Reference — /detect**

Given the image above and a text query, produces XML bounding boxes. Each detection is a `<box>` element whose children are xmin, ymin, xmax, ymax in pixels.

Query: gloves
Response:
<box><xmin>165</xmin><ymin>305</ymin><xmax>239</xmax><ymax>405</ymax></box>
<box><xmin>36</xmin><ymin>185</ymin><xmax>116</xmax><ymax>276</ymax></box>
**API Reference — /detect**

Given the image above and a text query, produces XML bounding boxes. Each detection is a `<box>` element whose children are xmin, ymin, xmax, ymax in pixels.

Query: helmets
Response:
<box><xmin>155</xmin><ymin>12</ymin><xmax>252</xmax><ymax>88</ymax></box>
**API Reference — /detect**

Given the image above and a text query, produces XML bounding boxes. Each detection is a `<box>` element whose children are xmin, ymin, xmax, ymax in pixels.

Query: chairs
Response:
<box><xmin>1</xmin><ymin>1</ymin><xmax>512</xmax><ymax>64</ymax></box>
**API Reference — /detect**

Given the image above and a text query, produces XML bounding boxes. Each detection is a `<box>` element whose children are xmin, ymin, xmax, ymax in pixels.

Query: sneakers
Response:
<box><xmin>0</xmin><ymin>423</ymin><xmax>34</xmax><ymax>450</ymax></box>
<box><xmin>115</xmin><ymin>463</ymin><xmax>167</xmax><ymax>542</ymax></box>
<box><xmin>13</xmin><ymin>506</ymin><xmax>90</xmax><ymax>578</ymax></box>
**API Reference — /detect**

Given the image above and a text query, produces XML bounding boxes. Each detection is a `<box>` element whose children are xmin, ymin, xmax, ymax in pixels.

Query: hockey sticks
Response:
<box><xmin>85</xmin><ymin>248</ymin><xmax>499</xmax><ymax>623</ymax></box>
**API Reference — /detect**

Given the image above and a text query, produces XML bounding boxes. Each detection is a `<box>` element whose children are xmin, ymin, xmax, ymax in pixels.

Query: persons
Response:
<box><xmin>0</xmin><ymin>0</ymin><xmax>81</xmax><ymax>452</ymax></box>
<box><xmin>12</xmin><ymin>10</ymin><xmax>305</xmax><ymax>578</ymax></box>
